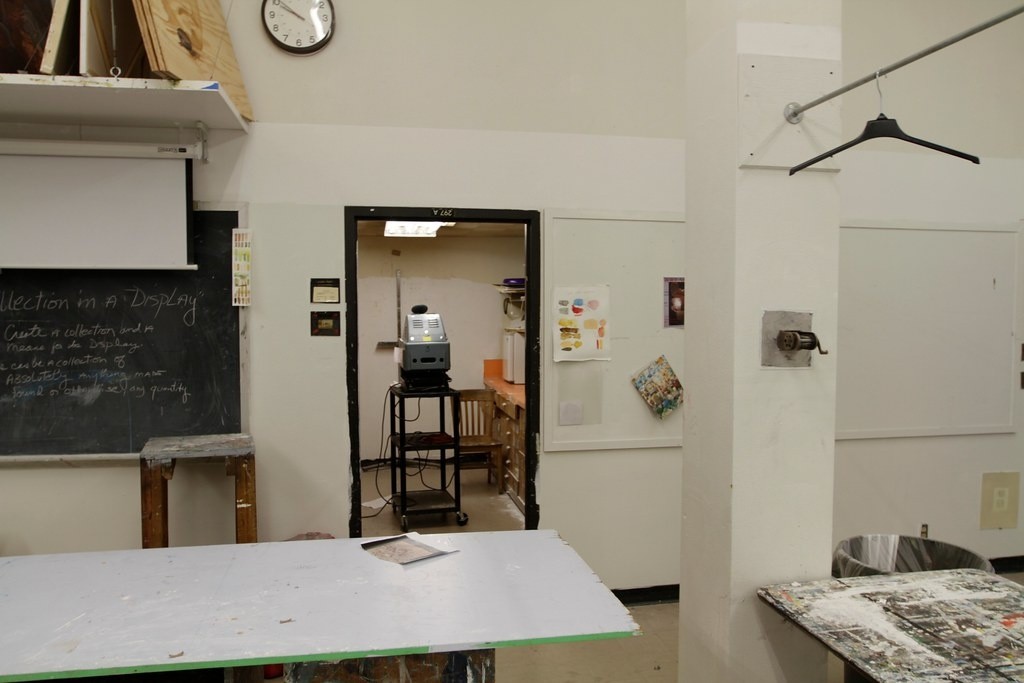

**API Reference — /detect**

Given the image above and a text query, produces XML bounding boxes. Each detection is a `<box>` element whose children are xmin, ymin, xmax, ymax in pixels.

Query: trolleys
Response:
<box><xmin>387</xmin><ymin>384</ymin><xmax>470</xmax><ymax>532</ymax></box>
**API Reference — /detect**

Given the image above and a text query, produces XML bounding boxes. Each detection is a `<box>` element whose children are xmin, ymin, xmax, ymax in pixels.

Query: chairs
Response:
<box><xmin>450</xmin><ymin>389</ymin><xmax>505</xmax><ymax>495</ymax></box>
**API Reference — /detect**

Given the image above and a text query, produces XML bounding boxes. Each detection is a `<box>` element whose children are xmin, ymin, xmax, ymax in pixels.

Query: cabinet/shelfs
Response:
<box><xmin>483</xmin><ymin>376</ymin><xmax>526</xmax><ymax>517</ymax></box>
<box><xmin>390</xmin><ymin>386</ymin><xmax>468</xmax><ymax>532</ymax></box>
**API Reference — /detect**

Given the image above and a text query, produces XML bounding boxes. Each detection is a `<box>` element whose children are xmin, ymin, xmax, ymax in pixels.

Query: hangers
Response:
<box><xmin>789</xmin><ymin>69</ymin><xmax>979</xmax><ymax>175</ymax></box>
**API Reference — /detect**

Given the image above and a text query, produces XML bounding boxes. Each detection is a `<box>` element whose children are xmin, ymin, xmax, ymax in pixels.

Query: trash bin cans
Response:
<box><xmin>837</xmin><ymin>533</ymin><xmax>993</xmax><ymax>683</ymax></box>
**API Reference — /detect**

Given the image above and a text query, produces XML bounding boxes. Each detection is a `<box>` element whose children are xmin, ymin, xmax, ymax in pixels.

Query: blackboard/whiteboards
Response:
<box><xmin>0</xmin><ymin>200</ymin><xmax>248</xmax><ymax>463</ymax></box>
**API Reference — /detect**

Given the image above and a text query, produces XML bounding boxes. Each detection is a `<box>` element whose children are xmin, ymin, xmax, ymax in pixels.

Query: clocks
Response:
<box><xmin>261</xmin><ymin>0</ymin><xmax>336</xmax><ymax>54</ymax></box>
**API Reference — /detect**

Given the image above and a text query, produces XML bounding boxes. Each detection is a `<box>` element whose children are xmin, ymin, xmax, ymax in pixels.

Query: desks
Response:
<box><xmin>0</xmin><ymin>527</ymin><xmax>644</xmax><ymax>683</ymax></box>
<box><xmin>758</xmin><ymin>569</ymin><xmax>1024</xmax><ymax>683</ymax></box>
